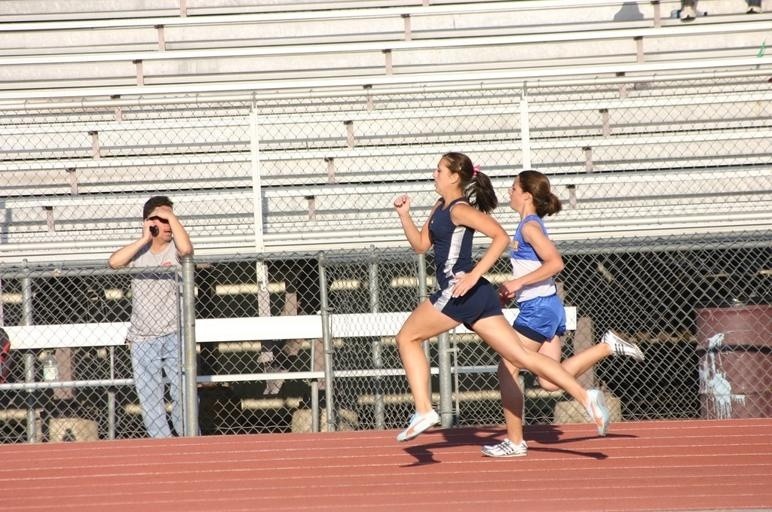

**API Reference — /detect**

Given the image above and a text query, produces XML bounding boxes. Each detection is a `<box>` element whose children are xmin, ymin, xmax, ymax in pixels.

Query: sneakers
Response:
<box><xmin>585</xmin><ymin>389</ymin><xmax>610</xmax><ymax>437</ymax></box>
<box><xmin>480</xmin><ymin>438</ymin><xmax>529</xmax><ymax>458</ymax></box>
<box><xmin>600</xmin><ymin>328</ymin><xmax>645</xmax><ymax>362</ymax></box>
<box><xmin>396</xmin><ymin>408</ymin><xmax>441</xmax><ymax>441</ymax></box>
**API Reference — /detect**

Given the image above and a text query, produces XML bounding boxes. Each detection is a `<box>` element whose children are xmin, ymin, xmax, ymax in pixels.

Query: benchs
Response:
<box><xmin>1</xmin><ymin>0</ymin><xmax>771</xmax><ymax>261</ymax></box>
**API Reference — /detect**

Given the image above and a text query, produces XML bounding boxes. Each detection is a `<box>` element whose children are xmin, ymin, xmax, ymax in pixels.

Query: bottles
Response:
<box><xmin>42</xmin><ymin>351</ymin><xmax>59</xmax><ymax>382</ymax></box>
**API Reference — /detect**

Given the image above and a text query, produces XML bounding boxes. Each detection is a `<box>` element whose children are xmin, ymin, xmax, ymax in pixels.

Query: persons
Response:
<box><xmin>480</xmin><ymin>169</ymin><xmax>646</xmax><ymax>459</ymax></box>
<box><xmin>392</xmin><ymin>151</ymin><xmax>611</xmax><ymax>443</ymax></box>
<box><xmin>106</xmin><ymin>195</ymin><xmax>201</xmax><ymax>438</ymax></box>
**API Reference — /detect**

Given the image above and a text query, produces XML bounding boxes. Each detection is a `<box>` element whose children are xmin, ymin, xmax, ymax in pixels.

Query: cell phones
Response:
<box><xmin>149</xmin><ymin>225</ymin><xmax>159</xmax><ymax>237</ymax></box>
